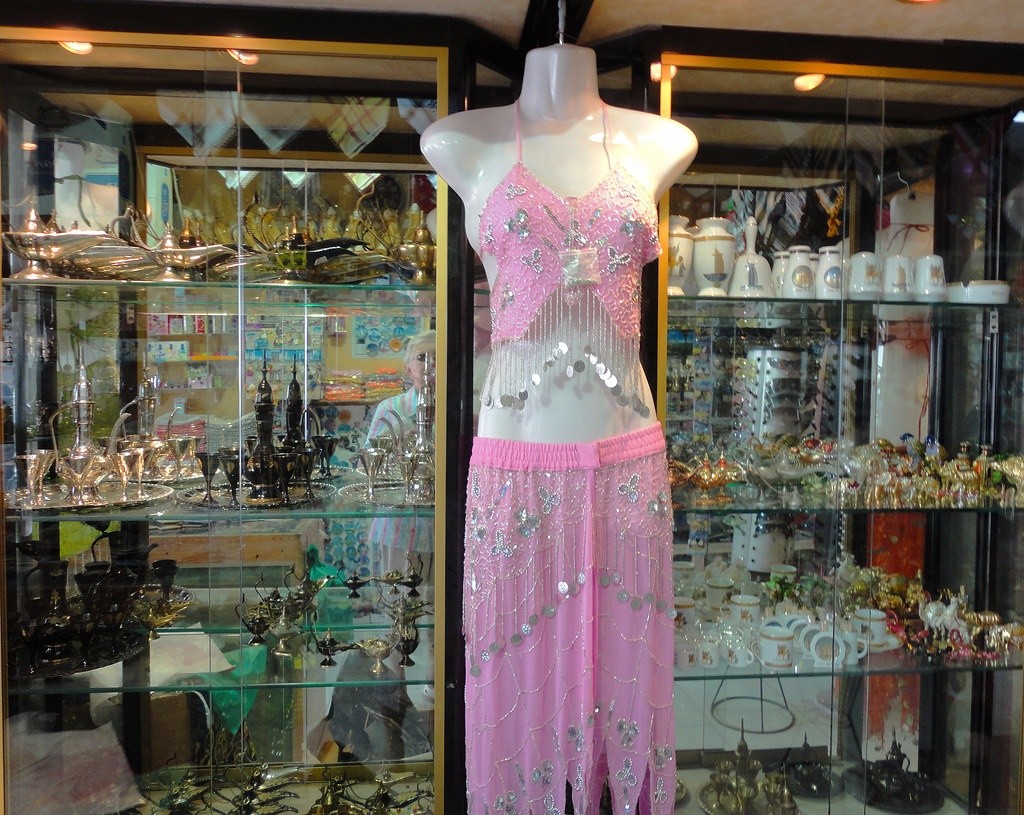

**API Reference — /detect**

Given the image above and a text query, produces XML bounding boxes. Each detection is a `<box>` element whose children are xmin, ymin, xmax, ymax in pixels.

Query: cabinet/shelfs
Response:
<box><xmin>584</xmin><ymin>22</ymin><xmax>1024</xmax><ymax>815</ymax></box>
<box><xmin>0</xmin><ymin>0</ymin><xmax>478</xmax><ymax>815</ymax></box>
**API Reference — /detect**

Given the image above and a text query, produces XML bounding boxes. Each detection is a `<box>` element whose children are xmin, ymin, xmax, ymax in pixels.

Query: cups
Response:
<box><xmin>673</xmin><ymin>596</ymin><xmax>696</xmax><ymax>625</ymax></box>
<box><xmin>360</xmin><ymin>434</ymin><xmax>415</xmax><ymax>505</ymax></box>
<box><xmin>852</xmin><ymin>608</ymin><xmax>887</xmax><ymax>647</ymax></box>
<box><xmin>842</xmin><ymin>632</ymin><xmax>867</xmax><ymax>665</ymax></box>
<box><xmin>770</xmin><ymin>564</ymin><xmax>797</xmax><ymax>585</ymax></box>
<box><xmin>847</xmin><ymin>251</ymin><xmax>946</xmax><ymax>302</ymax></box>
<box><xmin>674</xmin><ymin>622</ymin><xmax>754</xmax><ymax>669</ymax></box>
<box><xmin>751</xmin><ymin>626</ymin><xmax>794</xmax><ymax>669</ymax></box>
<box><xmin>719</xmin><ymin>595</ymin><xmax>761</xmax><ymax>622</ymax></box>
<box><xmin>700</xmin><ymin>578</ymin><xmax>735</xmax><ymax>609</ymax></box>
<box><xmin>672</xmin><ymin>561</ymin><xmax>696</xmax><ymax>586</ymax></box>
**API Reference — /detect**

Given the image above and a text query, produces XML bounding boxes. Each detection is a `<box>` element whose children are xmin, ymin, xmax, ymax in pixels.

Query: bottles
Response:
<box><xmin>26</xmin><ymin>561</ymin><xmax>81</xmax><ymax>673</ymax></box>
<box><xmin>771</xmin><ymin>244</ymin><xmax>846</xmax><ymax>299</ymax></box>
<box><xmin>669</xmin><ymin>214</ymin><xmax>736</xmax><ymax>296</ymax></box>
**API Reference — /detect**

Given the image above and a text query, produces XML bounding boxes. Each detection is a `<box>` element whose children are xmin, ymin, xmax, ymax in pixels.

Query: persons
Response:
<box><xmin>365</xmin><ymin>331</ymin><xmax>435</xmax><ymax>627</ymax></box>
<box><xmin>420</xmin><ymin>43</ymin><xmax>699</xmax><ymax>815</ymax></box>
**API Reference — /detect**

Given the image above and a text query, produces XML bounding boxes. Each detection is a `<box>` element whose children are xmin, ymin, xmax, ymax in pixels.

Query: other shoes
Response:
<box><xmin>422</xmin><ymin>685</ymin><xmax>434</xmax><ymax>704</ymax></box>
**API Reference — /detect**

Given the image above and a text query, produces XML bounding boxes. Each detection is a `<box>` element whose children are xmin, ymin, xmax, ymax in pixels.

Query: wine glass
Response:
<box><xmin>10</xmin><ymin>449</ymin><xmax>57</xmax><ymax>509</ymax></box>
<box><xmin>194</xmin><ymin>446</ymin><xmax>246</xmax><ymax>510</ymax></box>
<box><xmin>110</xmin><ymin>449</ymin><xmax>152</xmax><ymax>503</ymax></box>
<box><xmin>269</xmin><ymin>446</ymin><xmax>319</xmax><ymax>504</ymax></box>
<box><xmin>311</xmin><ymin>434</ymin><xmax>339</xmax><ymax>479</ymax></box>
<box><xmin>167</xmin><ymin>435</ymin><xmax>201</xmax><ymax>481</ymax></box>
<box><xmin>761</xmin><ymin>767</ymin><xmax>783</xmax><ymax>815</ymax></box>
<box><xmin>244</xmin><ymin>434</ymin><xmax>258</xmax><ymax>454</ymax></box>
<box><xmin>74</xmin><ymin>560</ymin><xmax>179</xmax><ymax>618</ymax></box>
<box><xmin>709</xmin><ymin>759</ymin><xmax>754</xmax><ymax>815</ymax></box>
<box><xmin>97</xmin><ymin>436</ymin><xmax>129</xmax><ymax>455</ymax></box>
<box><xmin>681</xmin><ymin>615</ymin><xmax>743</xmax><ymax>673</ymax></box>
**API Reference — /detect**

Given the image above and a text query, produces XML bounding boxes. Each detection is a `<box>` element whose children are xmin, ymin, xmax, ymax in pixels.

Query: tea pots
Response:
<box><xmin>235</xmin><ymin>552</ymin><xmax>434</xmax><ymax>675</ymax></box>
<box><xmin>794</xmin><ymin>733</ymin><xmax>819</xmax><ymax>782</ymax></box>
<box><xmin>119</xmin><ymin>367</ymin><xmax>181</xmax><ymax>475</ymax></box>
<box><xmin>138</xmin><ymin>751</ymin><xmax>433</xmax><ymax>815</ymax></box>
<box><xmin>729</xmin><ymin>718</ymin><xmax>761</xmax><ymax>805</ymax></box>
<box><xmin>86</xmin><ymin>562</ymin><xmax>137</xmax><ymax>657</ymax></box>
<box><xmin>90</xmin><ymin>529</ymin><xmax>159</xmax><ymax>591</ymax></box>
<box><xmin>408</xmin><ymin>351</ymin><xmax>436</xmax><ymax>503</ymax></box>
<box><xmin>49</xmin><ymin>364</ymin><xmax>131</xmax><ymax>504</ymax></box>
<box><xmin>883</xmin><ymin>728</ymin><xmax>910</xmax><ymax>795</ymax></box>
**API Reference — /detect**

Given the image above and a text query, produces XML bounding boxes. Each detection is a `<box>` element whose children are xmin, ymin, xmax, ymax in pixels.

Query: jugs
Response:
<box><xmin>274</xmin><ymin>354</ymin><xmax>314</xmax><ymax>480</ymax></box>
<box><xmin>245</xmin><ymin>349</ymin><xmax>289</xmax><ymax>504</ymax></box>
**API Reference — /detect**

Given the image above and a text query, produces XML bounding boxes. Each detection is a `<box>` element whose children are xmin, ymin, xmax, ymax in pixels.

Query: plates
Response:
<box><xmin>3</xmin><ymin>481</ymin><xmax>174</xmax><ymax>511</ymax></box>
<box><xmin>762</xmin><ymin>761</ymin><xmax>844</xmax><ymax>798</ymax></box>
<box><xmin>857</xmin><ymin>627</ymin><xmax>904</xmax><ymax>653</ymax></box>
<box><xmin>355</xmin><ymin>467</ymin><xmax>367</xmax><ymax>475</ymax></box>
<box><xmin>337</xmin><ymin>480</ymin><xmax>435</xmax><ymax>507</ymax></box>
<box><xmin>241</xmin><ymin>465</ymin><xmax>354</xmax><ymax>482</ymax></box>
<box><xmin>175</xmin><ymin>481</ymin><xmax>337</xmax><ymax>510</ymax></box>
<box><xmin>696</xmin><ymin>778</ymin><xmax>799</xmax><ymax>815</ymax></box>
<box><xmin>675</xmin><ymin>777</ymin><xmax>687</xmax><ymax>802</ymax></box>
<box><xmin>102</xmin><ymin>463</ymin><xmax>221</xmax><ymax>482</ymax></box>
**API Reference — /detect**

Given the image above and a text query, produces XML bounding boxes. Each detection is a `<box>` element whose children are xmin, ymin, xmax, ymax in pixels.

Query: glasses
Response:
<box><xmin>411</xmin><ymin>353</ymin><xmax>426</xmax><ymax>361</ymax></box>
